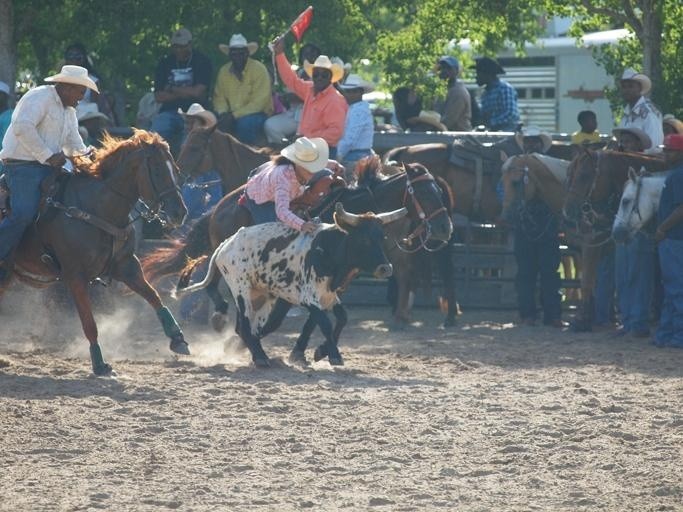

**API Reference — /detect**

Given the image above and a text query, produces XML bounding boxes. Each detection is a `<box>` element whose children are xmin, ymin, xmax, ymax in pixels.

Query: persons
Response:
<box><xmin>1</xmin><ymin>64</ymin><xmax>101</xmax><ymax>278</ymax></box>
<box><xmin>469</xmin><ymin>57</ymin><xmax>521</xmax><ymax>223</ymax></box>
<box><xmin>612</xmin><ymin>68</ymin><xmax>664</xmax><ymax>152</ymax></box>
<box><xmin>438</xmin><ymin>56</ymin><xmax>474</xmax><ymax>132</ymax></box>
<box><xmin>497</xmin><ymin>126</ymin><xmax>570</xmax><ymax>327</ymax></box>
<box><xmin>134</xmin><ymin>25</ymin><xmax>448</xmax><ymax>317</ymax></box>
<box><xmin>605</xmin><ymin>125</ymin><xmax>653</xmax><ymax>339</ymax></box>
<box><xmin>569</xmin><ymin>110</ymin><xmax>600</xmax><ymax>149</ymax></box>
<box><xmin>647</xmin><ymin>134</ymin><xmax>683</xmax><ymax>350</ymax></box>
<box><xmin>65</xmin><ymin>43</ymin><xmax>110</xmax><ymax>149</ymax></box>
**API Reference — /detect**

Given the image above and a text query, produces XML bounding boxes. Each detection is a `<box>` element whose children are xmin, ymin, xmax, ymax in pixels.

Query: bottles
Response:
<box><xmin>620</xmin><ymin>66</ymin><xmax>652</xmax><ymax>96</ymax></box>
<box><xmin>663</xmin><ymin>112</ymin><xmax>683</xmax><ymax>152</ymax></box>
<box><xmin>276</xmin><ymin>135</ymin><xmax>332</xmax><ymax>174</ymax></box>
<box><xmin>168</xmin><ymin>28</ymin><xmax>194</xmax><ymax>49</ymax></box>
<box><xmin>610</xmin><ymin>127</ymin><xmax>653</xmax><ymax>150</ymax></box>
<box><xmin>0</xmin><ymin>81</ymin><xmax>12</xmax><ymax>97</ymax></box>
<box><xmin>42</xmin><ymin>64</ymin><xmax>101</xmax><ymax>96</ymax></box>
<box><xmin>217</xmin><ymin>33</ymin><xmax>260</xmax><ymax>58</ymax></box>
<box><xmin>76</xmin><ymin>101</ymin><xmax>111</xmax><ymax>124</ymax></box>
<box><xmin>467</xmin><ymin>56</ymin><xmax>507</xmax><ymax>78</ymax></box>
<box><xmin>302</xmin><ymin>54</ymin><xmax>377</xmax><ymax>93</ymax></box>
<box><xmin>440</xmin><ymin>56</ymin><xmax>460</xmax><ymax>70</ymax></box>
<box><xmin>512</xmin><ymin>123</ymin><xmax>553</xmax><ymax>153</ymax></box>
<box><xmin>175</xmin><ymin>102</ymin><xmax>218</xmax><ymax>130</ymax></box>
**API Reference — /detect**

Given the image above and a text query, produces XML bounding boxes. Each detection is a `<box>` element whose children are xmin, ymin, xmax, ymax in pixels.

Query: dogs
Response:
<box><xmin>563</xmin><ymin>141</ymin><xmax>683</xmax><ymax>222</ymax></box>
<box><xmin>610</xmin><ymin>165</ymin><xmax>683</xmax><ymax>245</ymax></box>
<box><xmin>0</xmin><ymin>123</ymin><xmax>191</xmax><ymax>376</ymax></box>
<box><xmin>124</xmin><ymin>159</ymin><xmax>454</xmax><ymax>364</ymax></box>
<box><xmin>385</xmin><ymin>125</ymin><xmax>551</xmax><ymax>330</ymax></box>
<box><xmin>172</xmin><ymin>112</ymin><xmax>457</xmax><ymax>331</ymax></box>
<box><xmin>498</xmin><ymin>146</ymin><xmax>594</xmax><ymax>331</ymax></box>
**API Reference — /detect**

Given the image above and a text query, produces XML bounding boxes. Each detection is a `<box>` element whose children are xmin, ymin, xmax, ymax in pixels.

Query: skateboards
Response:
<box><xmin>176</xmin><ymin>200</ymin><xmax>409</xmax><ymax>368</ymax></box>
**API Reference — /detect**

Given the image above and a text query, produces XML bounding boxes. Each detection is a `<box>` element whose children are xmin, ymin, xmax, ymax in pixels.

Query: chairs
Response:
<box><xmin>607</xmin><ymin>323</ymin><xmax>683</xmax><ymax>349</ymax></box>
<box><xmin>519</xmin><ymin>317</ymin><xmax>571</xmax><ymax>331</ymax></box>
